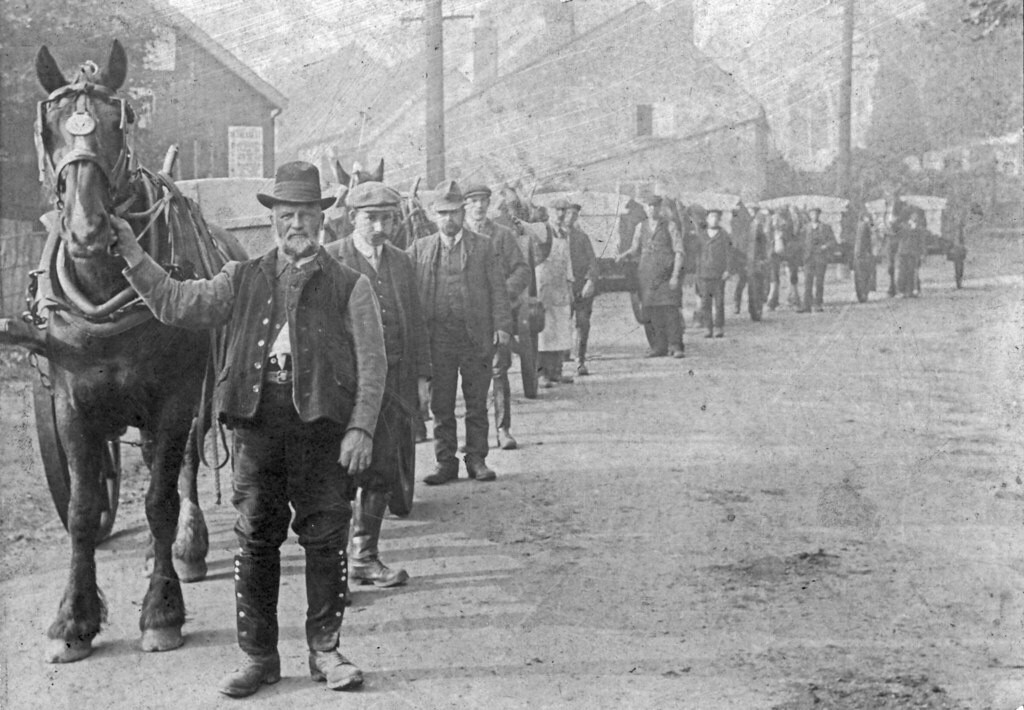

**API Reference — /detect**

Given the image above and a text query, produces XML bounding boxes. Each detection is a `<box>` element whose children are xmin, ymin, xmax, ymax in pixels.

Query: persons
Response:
<box><xmin>510</xmin><ymin>198</ymin><xmax>597</xmax><ymax>390</ymax></box>
<box><xmin>106</xmin><ymin>160</ymin><xmax>389</xmax><ymax>696</ymax></box>
<box><xmin>795</xmin><ymin>207</ymin><xmax>837</xmax><ymax>314</ymax></box>
<box><xmin>614</xmin><ymin>195</ymin><xmax>687</xmax><ymax>358</ymax></box>
<box><xmin>400</xmin><ymin>179</ymin><xmax>531</xmax><ymax>485</ymax></box>
<box><xmin>321</xmin><ymin>180</ymin><xmax>434</xmax><ymax>605</ymax></box>
<box><xmin>698</xmin><ymin>208</ymin><xmax>731</xmax><ymax>339</ymax></box>
<box><xmin>893</xmin><ymin>212</ymin><xmax>927</xmax><ymax>299</ymax></box>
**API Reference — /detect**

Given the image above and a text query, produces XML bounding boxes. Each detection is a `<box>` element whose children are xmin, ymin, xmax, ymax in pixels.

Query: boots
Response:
<box><xmin>345</xmin><ymin>484</ymin><xmax>408</xmax><ymax>587</ymax></box>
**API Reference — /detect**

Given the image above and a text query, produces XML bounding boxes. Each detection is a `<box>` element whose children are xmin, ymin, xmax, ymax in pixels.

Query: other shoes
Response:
<box><xmin>423</xmin><ymin>463</ymin><xmax>458</xmax><ymax>484</ymax></box>
<box><xmin>645</xmin><ymin>350</ymin><xmax>666</xmax><ymax>357</ymax></box>
<box><xmin>553</xmin><ymin>373</ymin><xmax>573</xmax><ymax>383</ymax></box>
<box><xmin>309</xmin><ymin>650</ymin><xmax>363</xmax><ymax>689</ymax></box>
<box><xmin>796</xmin><ymin>306</ymin><xmax>810</xmax><ymax>313</ymax></box>
<box><xmin>674</xmin><ymin>350</ymin><xmax>684</xmax><ymax>357</ymax></box>
<box><xmin>538</xmin><ymin>375</ymin><xmax>551</xmax><ymax>389</ymax></box>
<box><xmin>576</xmin><ymin>359</ymin><xmax>588</xmax><ymax>376</ymax></box>
<box><xmin>220</xmin><ymin>653</ymin><xmax>283</xmax><ymax>696</ymax></box>
<box><xmin>498</xmin><ymin>427</ymin><xmax>517</xmax><ymax>450</ymax></box>
<box><xmin>467</xmin><ymin>460</ymin><xmax>495</xmax><ymax>480</ymax></box>
<box><xmin>714</xmin><ymin>329</ymin><xmax>723</xmax><ymax>337</ymax></box>
<box><xmin>816</xmin><ymin>304</ymin><xmax>823</xmax><ymax>312</ymax></box>
<box><xmin>705</xmin><ymin>331</ymin><xmax>713</xmax><ymax>337</ymax></box>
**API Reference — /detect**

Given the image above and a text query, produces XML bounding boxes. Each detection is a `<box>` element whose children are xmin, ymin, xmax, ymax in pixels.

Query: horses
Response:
<box><xmin>762</xmin><ymin>200</ymin><xmax>813</xmax><ymax>314</ymax></box>
<box><xmin>882</xmin><ymin>184</ymin><xmax>924</xmax><ymax>300</ymax></box>
<box><xmin>487</xmin><ymin>176</ymin><xmax>551</xmax><ymax>229</ymax></box>
<box><xmin>315</xmin><ymin>158</ymin><xmax>445</xmax><ymax>446</ymax></box>
<box><xmin>656</xmin><ymin>191</ymin><xmax>707</xmax><ymax>332</ymax></box>
<box><xmin>31</xmin><ymin>37</ymin><xmax>250</xmax><ymax>667</ymax></box>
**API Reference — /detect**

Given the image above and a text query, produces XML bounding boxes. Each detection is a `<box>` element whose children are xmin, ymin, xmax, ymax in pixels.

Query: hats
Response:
<box><xmin>433</xmin><ymin>180</ymin><xmax>465</xmax><ymax>212</ymax></box>
<box><xmin>344</xmin><ymin>180</ymin><xmax>402</xmax><ymax>213</ymax></box>
<box><xmin>644</xmin><ymin>195</ymin><xmax>662</xmax><ymax>205</ymax></box>
<box><xmin>462</xmin><ymin>185</ymin><xmax>492</xmax><ymax>197</ymax></box>
<box><xmin>704</xmin><ymin>209</ymin><xmax>722</xmax><ymax>217</ymax></box>
<box><xmin>567</xmin><ymin>204</ymin><xmax>581</xmax><ymax>209</ymax></box>
<box><xmin>549</xmin><ymin>199</ymin><xmax>569</xmax><ymax>209</ymax></box>
<box><xmin>256</xmin><ymin>161</ymin><xmax>337</xmax><ymax>211</ymax></box>
